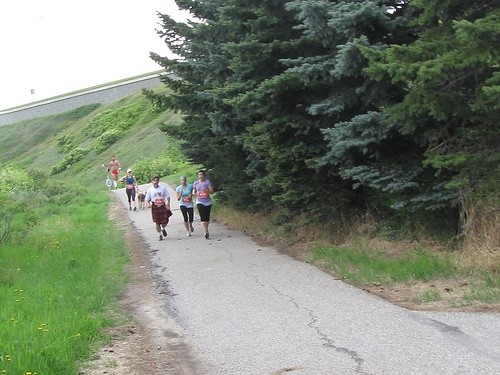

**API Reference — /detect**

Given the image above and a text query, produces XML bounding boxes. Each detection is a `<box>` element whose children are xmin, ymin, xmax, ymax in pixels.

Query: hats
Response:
<box><xmin>127</xmin><ymin>169</ymin><xmax>132</xmax><ymax>173</ymax></box>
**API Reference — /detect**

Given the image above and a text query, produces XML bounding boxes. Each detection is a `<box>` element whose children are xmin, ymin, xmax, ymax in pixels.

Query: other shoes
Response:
<box><xmin>158</xmin><ymin>235</ymin><xmax>163</xmax><ymax>241</ymax></box>
<box><xmin>134</xmin><ymin>207</ymin><xmax>136</xmax><ymax>211</ymax></box>
<box><xmin>162</xmin><ymin>229</ymin><xmax>167</xmax><ymax>237</ymax></box>
<box><xmin>205</xmin><ymin>231</ymin><xmax>209</xmax><ymax>239</ymax></box>
<box><xmin>189</xmin><ymin>226</ymin><xmax>194</xmax><ymax>232</ymax></box>
<box><xmin>129</xmin><ymin>207</ymin><xmax>131</xmax><ymax>210</ymax></box>
<box><xmin>187</xmin><ymin>231</ymin><xmax>191</xmax><ymax>236</ymax></box>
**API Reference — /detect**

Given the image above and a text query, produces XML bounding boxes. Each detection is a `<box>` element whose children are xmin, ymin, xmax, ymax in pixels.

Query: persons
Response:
<box><xmin>192</xmin><ymin>170</ymin><xmax>214</xmax><ymax>239</ymax></box>
<box><xmin>107</xmin><ymin>155</ymin><xmax>121</xmax><ymax>189</ymax></box>
<box><xmin>119</xmin><ymin>169</ymin><xmax>138</xmax><ymax>211</ymax></box>
<box><xmin>176</xmin><ymin>176</ymin><xmax>194</xmax><ymax>236</ymax></box>
<box><xmin>145</xmin><ymin>176</ymin><xmax>173</xmax><ymax>241</ymax></box>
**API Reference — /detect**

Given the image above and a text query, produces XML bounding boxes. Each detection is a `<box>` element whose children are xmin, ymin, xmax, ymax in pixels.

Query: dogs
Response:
<box><xmin>137</xmin><ymin>192</ymin><xmax>147</xmax><ymax>209</ymax></box>
